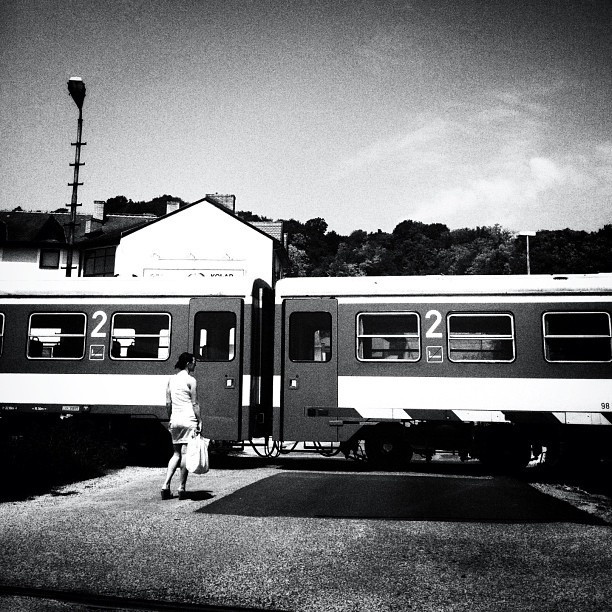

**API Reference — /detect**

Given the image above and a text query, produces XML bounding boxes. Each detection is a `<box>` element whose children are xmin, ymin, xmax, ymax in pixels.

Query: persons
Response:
<box><xmin>161</xmin><ymin>352</ymin><xmax>203</xmax><ymax>500</ymax></box>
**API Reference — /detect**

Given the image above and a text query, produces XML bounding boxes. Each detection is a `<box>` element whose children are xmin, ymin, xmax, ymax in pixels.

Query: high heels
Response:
<box><xmin>178</xmin><ymin>491</ymin><xmax>186</xmax><ymax>500</ymax></box>
<box><xmin>161</xmin><ymin>489</ymin><xmax>173</xmax><ymax>500</ymax></box>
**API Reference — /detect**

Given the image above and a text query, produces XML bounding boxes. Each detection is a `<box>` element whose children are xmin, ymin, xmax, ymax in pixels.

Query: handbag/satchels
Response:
<box><xmin>186</xmin><ymin>428</ymin><xmax>210</xmax><ymax>474</ymax></box>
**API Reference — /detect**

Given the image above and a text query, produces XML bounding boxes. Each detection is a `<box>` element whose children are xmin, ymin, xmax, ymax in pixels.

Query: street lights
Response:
<box><xmin>61</xmin><ymin>74</ymin><xmax>88</xmax><ymax>276</ymax></box>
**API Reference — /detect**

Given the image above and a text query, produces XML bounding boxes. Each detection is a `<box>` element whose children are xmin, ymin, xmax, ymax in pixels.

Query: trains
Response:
<box><xmin>0</xmin><ymin>271</ymin><xmax>612</xmax><ymax>470</ymax></box>
